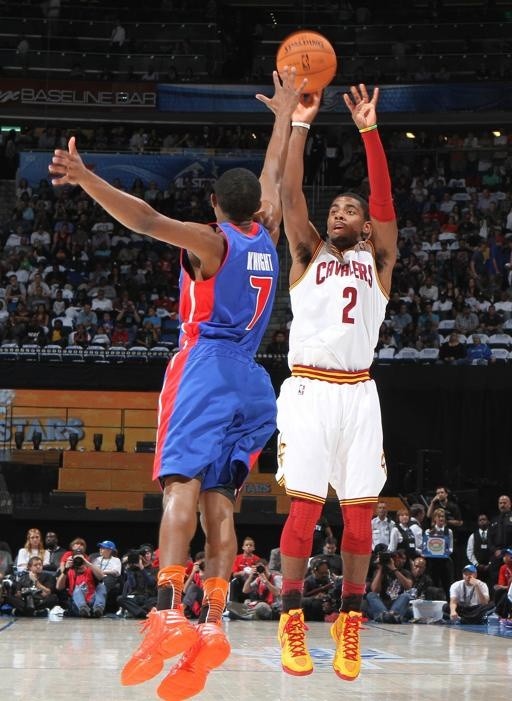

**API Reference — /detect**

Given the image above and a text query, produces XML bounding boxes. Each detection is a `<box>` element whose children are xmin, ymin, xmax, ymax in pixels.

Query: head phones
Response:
<box><xmin>143</xmin><ymin>544</ymin><xmax>154</xmax><ymax>560</ymax></box>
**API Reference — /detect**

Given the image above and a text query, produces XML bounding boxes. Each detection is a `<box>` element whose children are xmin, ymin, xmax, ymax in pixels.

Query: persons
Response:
<box><xmin>278</xmin><ymin>84</ymin><xmax>397</xmax><ymax>679</ymax></box>
<box><xmin>2</xmin><ymin>2</ymin><xmax>511</xmax><ymax>365</ymax></box>
<box><xmin>48</xmin><ymin>64</ymin><xmax>307</xmax><ymax>701</ymax></box>
<box><xmin>1</xmin><ymin>485</ymin><xmax>512</xmax><ymax>627</ymax></box>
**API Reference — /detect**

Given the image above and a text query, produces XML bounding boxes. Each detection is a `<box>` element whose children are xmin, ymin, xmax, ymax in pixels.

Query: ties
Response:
<box><xmin>482</xmin><ymin>532</ymin><xmax>485</xmax><ymax>542</ymax></box>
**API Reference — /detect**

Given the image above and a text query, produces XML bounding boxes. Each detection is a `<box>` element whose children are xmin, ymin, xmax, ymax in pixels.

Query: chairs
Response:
<box><xmin>1</xmin><ymin>181</ymin><xmax>213</xmax><ymax>367</ymax></box>
<box><xmin>370</xmin><ymin>174</ymin><xmax>511</xmax><ymax>360</ymax></box>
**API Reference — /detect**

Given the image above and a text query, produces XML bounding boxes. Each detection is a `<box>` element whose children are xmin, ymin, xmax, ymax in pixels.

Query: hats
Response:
<box><xmin>463</xmin><ymin>564</ymin><xmax>477</xmax><ymax>574</ymax></box>
<box><xmin>501</xmin><ymin>546</ymin><xmax>512</xmax><ymax>556</ymax></box>
<box><xmin>98</xmin><ymin>540</ymin><xmax>116</xmax><ymax>550</ymax></box>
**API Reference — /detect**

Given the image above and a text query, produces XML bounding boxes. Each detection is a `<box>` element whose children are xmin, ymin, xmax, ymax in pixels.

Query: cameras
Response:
<box><xmin>199</xmin><ymin>561</ymin><xmax>206</xmax><ymax>571</ymax></box>
<box><xmin>22</xmin><ymin>589</ymin><xmax>35</xmax><ymax>613</ymax></box>
<box><xmin>73</xmin><ymin>557</ymin><xmax>83</xmax><ymax>568</ymax></box>
<box><xmin>257</xmin><ymin>566</ymin><xmax>264</xmax><ymax>573</ymax></box>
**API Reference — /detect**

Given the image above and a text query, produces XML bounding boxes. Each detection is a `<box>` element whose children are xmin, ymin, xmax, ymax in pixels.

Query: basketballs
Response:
<box><xmin>277</xmin><ymin>32</ymin><xmax>337</xmax><ymax>94</ymax></box>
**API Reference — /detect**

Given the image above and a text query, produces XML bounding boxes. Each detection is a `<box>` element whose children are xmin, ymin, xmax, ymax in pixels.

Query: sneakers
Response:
<box><xmin>80</xmin><ymin>605</ymin><xmax>103</xmax><ymax>618</ymax></box>
<box><xmin>12</xmin><ymin>608</ymin><xmax>50</xmax><ymax>618</ymax></box>
<box><xmin>377</xmin><ymin>611</ymin><xmax>403</xmax><ymax>624</ymax></box>
<box><xmin>121</xmin><ymin>607</ymin><xmax>197</xmax><ymax>687</ymax></box>
<box><xmin>278</xmin><ymin>607</ymin><xmax>314</xmax><ymax>676</ymax></box>
<box><xmin>330</xmin><ymin>611</ymin><xmax>367</xmax><ymax>681</ymax></box>
<box><xmin>49</xmin><ymin>605</ymin><xmax>67</xmax><ymax>614</ymax></box>
<box><xmin>157</xmin><ymin>622</ymin><xmax>231</xmax><ymax>701</ymax></box>
